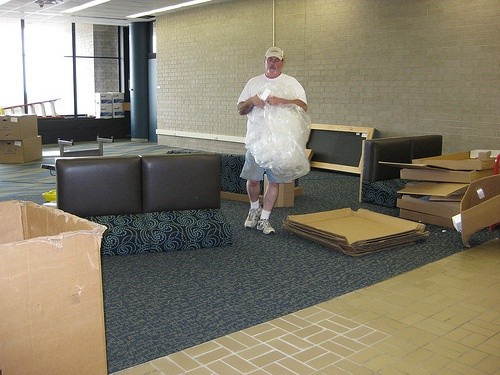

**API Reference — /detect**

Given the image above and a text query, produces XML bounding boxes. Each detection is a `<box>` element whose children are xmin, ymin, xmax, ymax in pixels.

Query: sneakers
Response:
<box><xmin>244</xmin><ymin>205</ymin><xmax>262</xmax><ymax>229</ymax></box>
<box><xmin>257</xmin><ymin>219</ymin><xmax>275</xmax><ymax>234</ymax></box>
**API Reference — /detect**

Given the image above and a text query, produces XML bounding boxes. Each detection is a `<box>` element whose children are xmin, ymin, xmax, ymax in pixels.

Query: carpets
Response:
<box><xmin>0</xmin><ymin>139</ymin><xmax>500</xmax><ymax>375</ymax></box>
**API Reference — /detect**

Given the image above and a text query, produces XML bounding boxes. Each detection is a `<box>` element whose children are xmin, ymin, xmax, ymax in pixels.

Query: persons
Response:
<box><xmin>236</xmin><ymin>46</ymin><xmax>307</xmax><ymax>234</ymax></box>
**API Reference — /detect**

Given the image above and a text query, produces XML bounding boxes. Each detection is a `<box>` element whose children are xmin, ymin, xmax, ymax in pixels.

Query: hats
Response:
<box><xmin>265</xmin><ymin>47</ymin><xmax>284</xmax><ymax>60</ymax></box>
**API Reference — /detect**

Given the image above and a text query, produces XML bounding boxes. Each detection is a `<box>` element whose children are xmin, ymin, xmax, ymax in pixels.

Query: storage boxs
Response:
<box><xmin>379</xmin><ymin>151</ymin><xmax>496</xmax><ymax>227</ymax></box>
<box><xmin>0</xmin><ymin>201</ymin><xmax>109</xmax><ymax>375</ymax></box>
<box><xmin>0</xmin><ymin>115</ymin><xmax>42</xmax><ymax>164</ymax></box>
<box><xmin>95</xmin><ymin>92</ymin><xmax>125</xmax><ymax>119</ymax></box>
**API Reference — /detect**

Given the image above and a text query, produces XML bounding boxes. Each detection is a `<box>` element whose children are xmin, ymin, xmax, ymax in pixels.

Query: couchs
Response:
<box><xmin>220</xmin><ymin>154</ymin><xmax>299</xmax><ymax>195</ymax></box>
<box><xmin>362</xmin><ymin>135</ymin><xmax>442</xmax><ymax>208</ymax></box>
<box><xmin>56</xmin><ymin>154</ymin><xmax>234</xmax><ymax>248</ymax></box>
